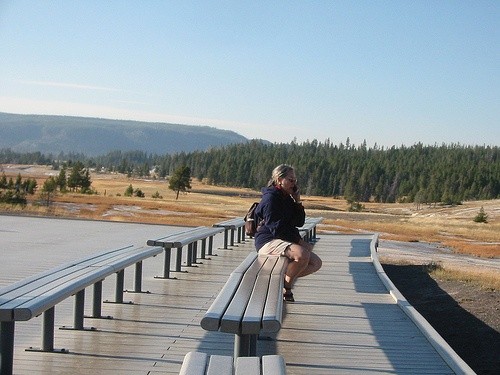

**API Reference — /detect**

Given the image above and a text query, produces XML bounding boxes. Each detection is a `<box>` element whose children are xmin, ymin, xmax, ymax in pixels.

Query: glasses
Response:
<box><xmin>283</xmin><ymin>177</ymin><xmax>298</xmax><ymax>184</ymax></box>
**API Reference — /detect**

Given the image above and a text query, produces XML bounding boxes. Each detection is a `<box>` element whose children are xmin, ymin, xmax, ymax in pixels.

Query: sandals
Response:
<box><xmin>282</xmin><ymin>287</ymin><xmax>296</xmax><ymax>303</ymax></box>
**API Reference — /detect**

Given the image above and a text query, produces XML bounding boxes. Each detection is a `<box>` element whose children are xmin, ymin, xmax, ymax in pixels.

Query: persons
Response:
<box><xmin>254</xmin><ymin>164</ymin><xmax>322</xmax><ymax>302</ymax></box>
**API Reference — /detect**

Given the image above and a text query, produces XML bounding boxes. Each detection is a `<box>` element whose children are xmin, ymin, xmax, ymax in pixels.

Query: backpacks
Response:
<box><xmin>243</xmin><ymin>201</ymin><xmax>260</xmax><ymax>236</ymax></box>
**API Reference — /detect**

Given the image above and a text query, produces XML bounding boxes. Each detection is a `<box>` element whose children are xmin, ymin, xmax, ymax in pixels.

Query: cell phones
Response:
<box><xmin>293</xmin><ymin>186</ymin><xmax>299</xmax><ymax>193</ymax></box>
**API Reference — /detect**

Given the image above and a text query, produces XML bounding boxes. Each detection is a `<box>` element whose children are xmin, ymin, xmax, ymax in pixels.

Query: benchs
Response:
<box><xmin>179</xmin><ymin>352</ymin><xmax>286</xmax><ymax>375</ymax></box>
<box><xmin>0</xmin><ymin>243</ymin><xmax>164</xmax><ymax>375</ymax></box>
<box><xmin>201</xmin><ymin>252</ymin><xmax>289</xmax><ymax>364</ymax></box>
<box><xmin>146</xmin><ymin>226</ymin><xmax>225</xmax><ymax>280</ymax></box>
<box><xmin>296</xmin><ymin>217</ymin><xmax>324</xmax><ymax>248</ymax></box>
<box><xmin>213</xmin><ymin>217</ymin><xmax>249</xmax><ymax>250</ymax></box>
